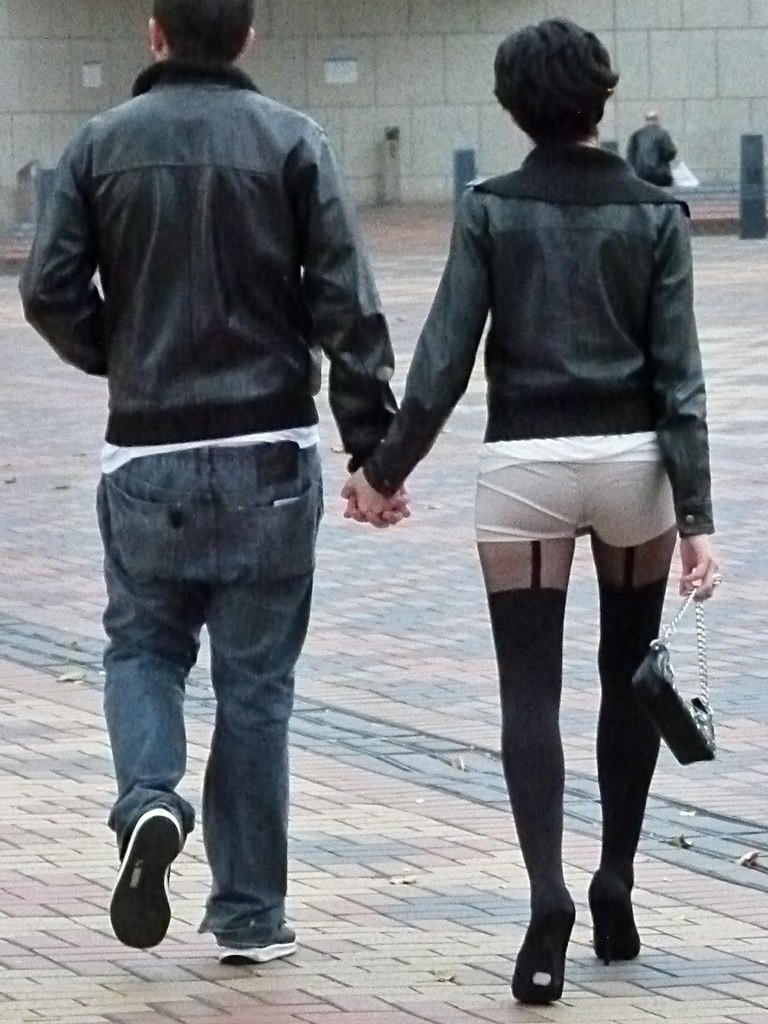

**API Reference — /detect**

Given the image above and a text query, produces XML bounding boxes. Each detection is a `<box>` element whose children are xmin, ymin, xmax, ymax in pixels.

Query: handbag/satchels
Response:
<box><xmin>631</xmin><ymin>587</ymin><xmax>716</xmax><ymax>765</ymax></box>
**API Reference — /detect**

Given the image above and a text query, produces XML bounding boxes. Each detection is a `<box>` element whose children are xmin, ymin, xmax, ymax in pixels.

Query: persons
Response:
<box><xmin>627</xmin><ymin>109</ymin><xmax>677</xmax><ymax>188</ymax></box>
<box><xmin>341</xmin><ymin>18</ymin><xmax>722</xmax><ymax>1004</ymax></box>
<box><xmin>16</xmin><ymin>0</ymin><xmax>410</xmax><ymax>965</ymax></box>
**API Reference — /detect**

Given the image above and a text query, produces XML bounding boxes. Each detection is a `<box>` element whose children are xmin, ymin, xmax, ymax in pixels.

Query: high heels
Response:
<box><xmin>512</xmin><ymin>885</ymin><xmax>576</xmax><ymax>1003</ymax></box>
<box><xmin>589</xmin><ymin>869</ymin><xmax>640</xmax><ymax>966</ymax></box>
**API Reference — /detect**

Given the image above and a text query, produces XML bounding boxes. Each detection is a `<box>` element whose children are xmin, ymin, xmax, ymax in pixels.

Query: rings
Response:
<box><xmin>713</xmin><ymin>574</ymin><xmax>722</xmax><ymax>586</ymax></box>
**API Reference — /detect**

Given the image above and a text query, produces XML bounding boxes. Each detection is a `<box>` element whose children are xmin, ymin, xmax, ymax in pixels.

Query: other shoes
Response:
<box><xmin>109</xmin><ymin>808</ymin><xmax>182</xmax><ymax>948</ymax></box>
<box><xmin>216</xmin><ymin>920</ymin><xmax>297</xmax><ymax>964</ymax></box>
